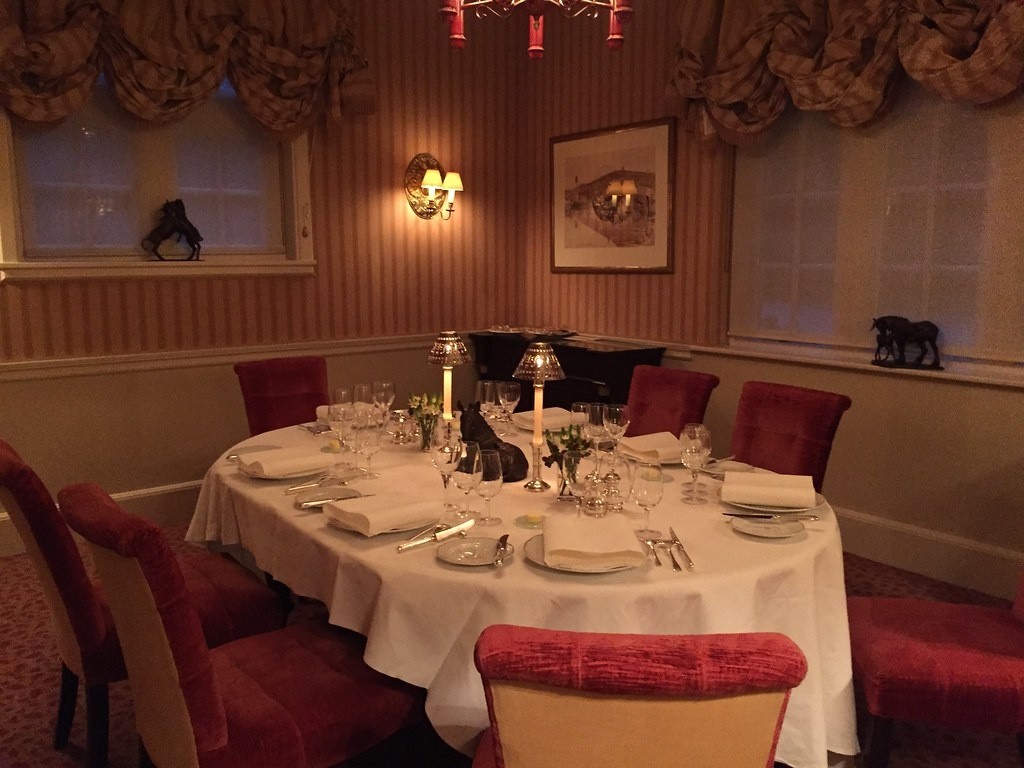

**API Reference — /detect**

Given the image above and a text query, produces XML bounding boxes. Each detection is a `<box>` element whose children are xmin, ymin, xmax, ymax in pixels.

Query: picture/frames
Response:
<box><xmin>549</xmin><ymin>115</ymin><xmax>679</xmax><ymax>275</ymax></box>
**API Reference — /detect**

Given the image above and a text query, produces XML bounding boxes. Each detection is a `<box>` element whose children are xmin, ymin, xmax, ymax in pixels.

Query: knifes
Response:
<box><xmin>494</xmin><ymin>535</ymin><xmax>511</xmax><ymax>565</ymax></box>
<box><xmin>669</xmin><ymin>526</ymin><xmax>694</xmax><ymax>572</ymax></box>
<box><xmin>397</xmin><ymin>519</ymin><xmax>476</xmax><ymax>552</ymax></box>
<box><xmin>300</xmin><ymin>495</ymin><xmax>375</xmax><ymax>509</ymax></box>
<box><xmin>722</xmin><ymin>513</ymin><xmax>819</xmax><ymax>522</ymax></box>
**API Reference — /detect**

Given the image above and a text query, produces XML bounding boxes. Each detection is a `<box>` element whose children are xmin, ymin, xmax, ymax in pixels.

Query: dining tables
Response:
<box><xmin>182</xmin><ymin>414</ymin><xmax>860</xmax><ymax>768</ymax></box>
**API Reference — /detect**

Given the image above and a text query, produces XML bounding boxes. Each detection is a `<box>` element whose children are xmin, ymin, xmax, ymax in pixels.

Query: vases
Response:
<box><xmin>554</xmin><ymin>458</ymin><xmax>579</xmax><ymax>500</ymax></box>
<box><xmin>416</xmin><ymin>417</ymin><xmax>437</xmax><ymax>452</ymax></box>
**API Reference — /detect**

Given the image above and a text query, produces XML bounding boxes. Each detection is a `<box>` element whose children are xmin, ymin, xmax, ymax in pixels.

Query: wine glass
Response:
<box><xmin>564</xmin><ymin>400</ymin><xmax>664</xmax><ymax>539</ymax></box>
<box><xmin>326</xmin><ymin>379</ymin><xmax>521</xmax><ymax>527</ymax></box>
<box><xmin>679</xmin><ymin>423</ymin><xmax>712</xmax><ymax>505</ymax></box>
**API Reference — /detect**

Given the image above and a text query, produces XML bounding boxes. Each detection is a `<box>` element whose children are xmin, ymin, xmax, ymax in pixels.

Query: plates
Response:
<box><xmin>524</xmin><ymin>534</ymin><xmax>652</xmax><ymax>574</ymax></box>
<box><xmin>699</xmin><ymin>459</ymin><xmax>755</xmax><ymax>476</ymax></box>
<box><xmin>296</xmin><ymin>488</ymin><xmax>361</xmax><ymax>503</ymax></box>
<box><xmin>237</xmin><ymin>468</ymin><xmax>328</xmax><ymax>479</ymax></box>
<box><xmin>717</xmin><ymin>487</ymin><xmax>826</xmax><ymax>512</ymax></box>
<box><xmin>437</xmin><ymin>537</ymin><xmax>514</xmax><ymax>567</ymax></box>
<box><xmin>328</xmin><ymin>520</ymin><xmax>436</xmax><ymax>534</ymax></box>
<box><xmin>659</xmin><ymin>458</ymin><xmax>683</xmax><ymax>466</ymax></box>
<box><xmin>515</xmin><ymin>424</ymin><xmax>574</xmax><ymax>433</ymax></box>
<box><xmin>731</xmin><ymin>513</ymin><xmax>805</xmax><ymax>537</ymax></box>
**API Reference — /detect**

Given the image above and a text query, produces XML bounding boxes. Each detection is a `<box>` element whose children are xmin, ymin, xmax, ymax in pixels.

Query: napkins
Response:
<box><xmin>234</xmin><ymin>399</ymin><xmax>815</xmax><ymax>574</ymax></box>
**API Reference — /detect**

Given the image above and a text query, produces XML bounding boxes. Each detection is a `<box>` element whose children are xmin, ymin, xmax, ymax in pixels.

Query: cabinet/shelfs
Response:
<box><xmin>467</xmin><ymin>328</ymin><xmax>665</xmax><ymax>408</ymax></box>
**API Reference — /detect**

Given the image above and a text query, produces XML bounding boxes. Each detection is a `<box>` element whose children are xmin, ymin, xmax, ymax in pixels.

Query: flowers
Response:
<box><xmin>408</xmin><ymin>392</ymin><xmax>442</xmax><ymax>448</ymax></box>
<box><xmin>541</xmin><ymin>423</ymin><xmax>594</xmax><ymax>496</ymax></box>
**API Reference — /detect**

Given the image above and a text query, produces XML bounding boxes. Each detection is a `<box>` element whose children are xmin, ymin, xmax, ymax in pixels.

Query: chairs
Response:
<box><xmin>618</xmin><ymin>363</ymin><xmax>721</xmax><ymax>440</ymax></box>
<box><xmin>56</xmin><ymin>479</ymin><xmax>426</xmax><ymax>768</ymax></box>
<box><xmin>468</xmin><ymin>623</ymin><xmax>808</xmax><ymax>768</ymax></box>
<box><xmin>232</xmin><ymin>356</ymin><xmax>329</xmax><ymax>439</ymax></box>
<box><xmin>844</xmin><ymin>572</ymin><xmax>1024</xmax><ymax>768</ymax></box>
<box><xmin>1</xmin><ymin>435</ymin><xmax>283</xmax><ymax>768</ymax></box>
<box><xmin>728</xmin><ymin>381</ymin><xmax>852</xmax><ymax>495</ymax></box>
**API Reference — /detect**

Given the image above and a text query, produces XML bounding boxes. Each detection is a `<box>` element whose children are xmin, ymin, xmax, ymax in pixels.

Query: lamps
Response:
<box><xmin>512</xmin><ymin>339</ymin><xmax>566</xmax><ymax>490</ymax></box>
<box><xmin>403</xmin><ymin>151</ymin><xmax>464</xmax><ymax>221</ymax></box>
<box><xmin>426</xmin><ymin>329</ymin><xmax>472</xmax><ymax>455</ymax></box>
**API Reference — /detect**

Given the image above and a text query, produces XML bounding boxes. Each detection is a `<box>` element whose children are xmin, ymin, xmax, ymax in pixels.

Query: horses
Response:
<box><xmin>869</xmin><ymin>316</ymin><xmax>940</xmax><ymax>368</ymax></box>
<box><xmin>142</xmin><ymin>199</ymin><xmax>204</xmax><ymax>260</ymax></box>
<box><xmin>453</xmin><ymin>400</ymin><xmax>528</xmax><ymax>483</ymax></box>
